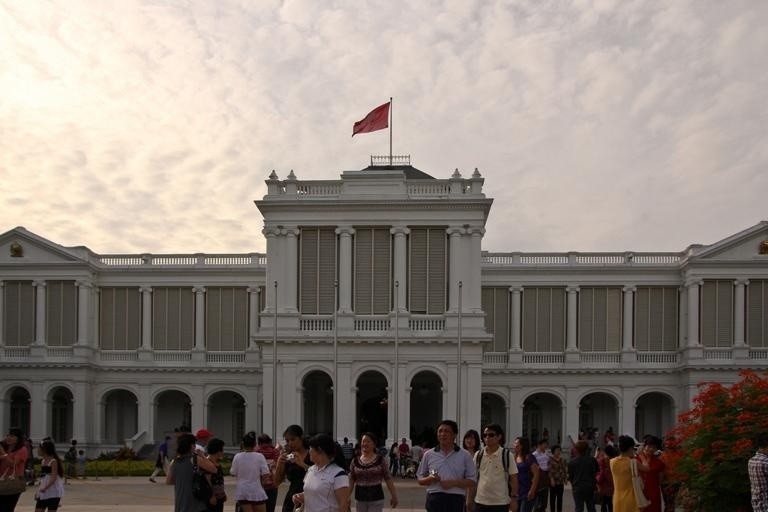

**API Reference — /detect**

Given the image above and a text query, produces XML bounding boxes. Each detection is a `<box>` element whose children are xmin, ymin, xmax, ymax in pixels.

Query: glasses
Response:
<box><xmin>481</xmin><ymin>434</ymin><xmax>495</xmax><ymax>438</ymax></box>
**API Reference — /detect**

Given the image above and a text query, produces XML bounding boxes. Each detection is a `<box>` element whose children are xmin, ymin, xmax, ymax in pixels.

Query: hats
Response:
<box><xmin>196</xmin><ymin>430</ymin><xmax>213</xmax><ymax>439</ymax></box>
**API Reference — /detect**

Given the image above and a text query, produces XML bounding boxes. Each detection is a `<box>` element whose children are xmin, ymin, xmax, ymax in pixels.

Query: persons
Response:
<box><xmin>511</xmin><ymin>436</ymin><xmax>567</xmax><ymax>511</ymax></box>
<box><xmin>748</xmin><ymin>439</ymin><xmax>768</xmax><ymax>511</ymax></box>
<box><xmin>149</xmin><ymin>428</ymin><xmax>227</xmax><ymax>511</ymax></box>
<box><xmin>0</xmin><ymin>427</ymin><xmax>27</xmax><ymax>512</ymax></box>
<box><xmin>276</xmin><ymin>425</ymin><xmax>399</xmax><ymax>511</ymax></box>
<box><xmin>466</xmin><ymin>423</ymin><xmax>519</xmax><ymax>511</ymax></box>
<box><xmin>463</xmin><ymin>430</ymin><xmax>479</xmax><ymax>454</ymax></box>
<box><xmin>568</xmin><ymin>427</ymin><xmax>679</xmax><ymax>512</ymax></box>
<box><xmin>389</xmin><ymin>438</ymin><xmax>432</xmax><ymax>479</ymax></box>
<box><xmin>35</xmin><ymin>439</ymin><xmax>63</xmax><ymax>512</ymax></box>
<box><xmin>230</xmin><ymin>432</ymin><xmax>281</xmax><ymax>511</ymax></box>
<box><xmin>416</xmin><ymin>420</ymin><xmax>476</xmax><ymax>511</ymax></box>
<box><xmin>68</xmin><ymin>439</ymin><xmax>78</xmax><ymax>478</ymax></box>
<box><xmin>78</xmin><ymin>449</ymin><xmax>87</xmax><ymax>478</ymax></box>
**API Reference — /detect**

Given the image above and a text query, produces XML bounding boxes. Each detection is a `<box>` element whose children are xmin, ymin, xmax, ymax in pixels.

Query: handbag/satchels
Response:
<box><xmin>0</xmin><ymin>474</ymin><xmax>26</xmax><ymax>495</ymax></box>
<box><xmin>191</xmin><ymin>471</ymin><xmax>212</xmax><ymax>502</ymax></box>
<box><xmin>631</xmin><ymin>476</ymin><xmax>650</xmax><ymax>509</ymax></box>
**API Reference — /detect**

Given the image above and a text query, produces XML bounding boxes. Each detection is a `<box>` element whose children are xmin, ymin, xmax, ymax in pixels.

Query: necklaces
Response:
<box><xmin>24</xmin><ymin>434</ymin><xmax>36</xmax><ymax>480</ymax></box>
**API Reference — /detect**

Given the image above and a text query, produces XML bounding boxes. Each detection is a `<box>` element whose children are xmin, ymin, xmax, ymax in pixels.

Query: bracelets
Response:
<box><xmin>511</xmin><ymin>495</ymin><xmax>518</xmax><ymax>498</ymax></box>
<box><xmin>4</xmin><ymin>453</ymin><xmax>8</xmax><ymax>458</ymax></box>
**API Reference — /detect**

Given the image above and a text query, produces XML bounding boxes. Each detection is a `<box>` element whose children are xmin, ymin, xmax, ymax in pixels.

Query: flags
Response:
<box><xmin>352</xmin><ymin>101</ymin><xmax>390</xmax><ymax>137</ymax></box>
